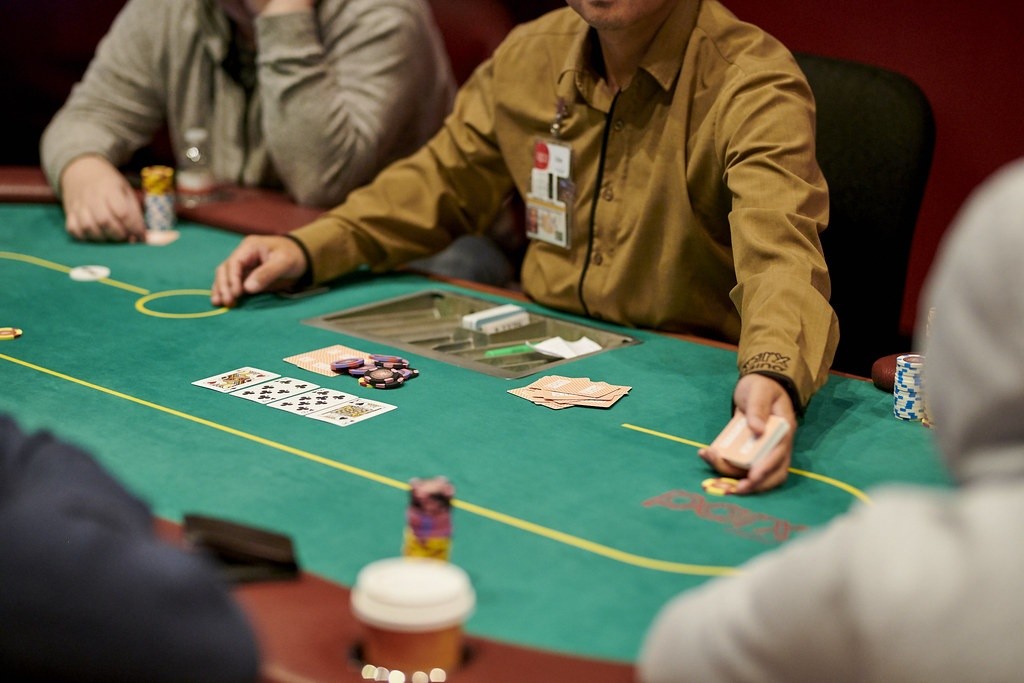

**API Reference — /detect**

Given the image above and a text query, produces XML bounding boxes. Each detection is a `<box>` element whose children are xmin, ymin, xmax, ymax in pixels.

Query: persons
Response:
<box><xmin>42</xmin><ymin>0</ymin><xmax>458</xmax><ymax>241</ymax></box>
<box><xmin>636</xmin><ymin>159</ymin><xmax>1024</xmax><ymax>683</ymax></box>
<box><xmin>210</xmin><ymin>0</ymin><xmax>840</xmax><ymax>491</ymax></box>
<box><xmin>1</xmin><ymin>411</ymin><xmax>261</xmax><ymax>683</ymax></box>
<box><xmin>527</xmin><ymin>208</ymin><xmax>537</xmax><ymax>234</ymax></box>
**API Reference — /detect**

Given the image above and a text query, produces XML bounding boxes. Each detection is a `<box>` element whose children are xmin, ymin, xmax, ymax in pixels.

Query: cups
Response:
<box><xmin>349</xmin><ymin>557</ymin><xmax>476</xmax><ymax>681</ymax></box>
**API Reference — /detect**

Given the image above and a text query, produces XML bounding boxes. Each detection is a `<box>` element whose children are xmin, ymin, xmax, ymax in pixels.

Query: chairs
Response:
<box><xmin>794</xmin><ymin>51</ymin><xmax>938</xmax><ymax>372</ymax></box>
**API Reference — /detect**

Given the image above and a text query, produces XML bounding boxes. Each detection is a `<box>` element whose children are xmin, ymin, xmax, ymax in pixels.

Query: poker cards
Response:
<box><xmin>229</xmin><ymin>377</ymin><xmax>320</xmax><ymax>404</ymax></box>
<box><xmin>708</xmin><ymin>409</ymin><xmax>789</xmax><ymax>469</ymax></box>
<box><xmin>282</xmin><ymin>344</ymin><xmax>376</xmax><ymax>378</ymax></box>
<box><xmin>266</xmin><ymin>388</ymin><xmax>358</xmax><ymax>416</ymax></box>
<box><xmin>506</xmin><ymin>373</ymin><xmax>633</xmax><ymax>410</ymax></box>
<box><xmin>190</xmin><ymin>366</ymin><xmax>282</xmax><ymax>392</ymax></box>
<box><xmin>306</xmin><ymin>397</ymin><xmax>398</xmax><ymax>427</ymax></box>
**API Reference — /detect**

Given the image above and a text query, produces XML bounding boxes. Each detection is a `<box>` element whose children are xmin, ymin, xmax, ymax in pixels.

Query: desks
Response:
<box><xmin>0</xmin><ymin>164</ymin><xmax>952</xmax><ymax>683</ymax></box>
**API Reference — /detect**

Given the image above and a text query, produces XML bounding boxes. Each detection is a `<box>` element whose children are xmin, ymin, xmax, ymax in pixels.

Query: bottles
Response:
<box><xmin>176</xmin><ymin>127</ymin><xmax>215</xmax><ymax>207</ymax></box>
